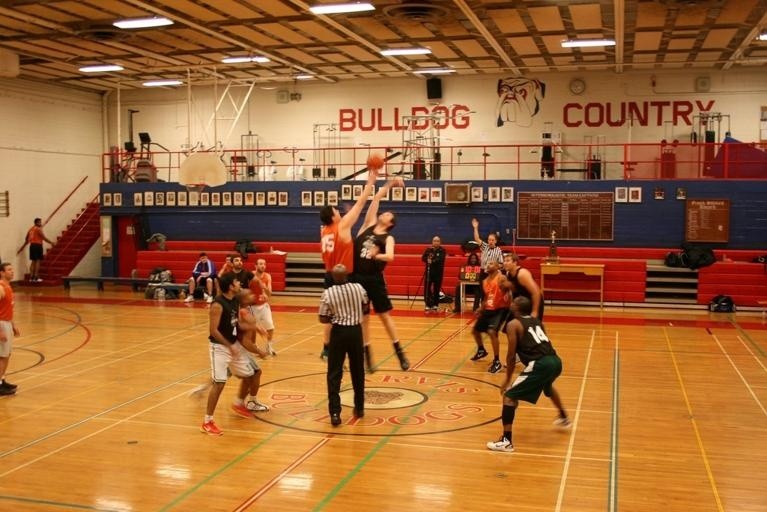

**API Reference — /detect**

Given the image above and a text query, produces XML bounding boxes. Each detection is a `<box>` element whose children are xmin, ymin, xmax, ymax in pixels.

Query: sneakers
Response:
<box><xmin>245</xmin><ymin>399</ymin><xmax>271</xmax><ymax>413</ymax></box>
<box><xmin>0</xmin><ymin>376</ymin><xmax>18</xmax><ymax>397</ymax></box>
<box><xmin>205</xmin><ymin>296</ymin><xmax>213</xmax><ymax>304</ymax></box>
<box><xmin>228</xmin><ymin>401</ymin><xmax>255</xmax><ymax>420</ymax></box>
<box><xmin>487</xmin><ymin>359</ymin><xmax>503</xmax><ymax>375</ymax></box>
<box><xmin>424</xmin><ymin>305</ymin><xmax>439</xmax><ymax>311</ymax></box>
<box><xmin>198</xmin><ymin>419</ymin><xmax>225</xmax><ymax>439</ymax></box>
<box><xmin>183</xmin><ymin>294</ymin><xmax>195</xmax><ymax>303</ymax></box>
<box><xmin>266</xmin><ymin>343</ymin><xmax>278</xmax><ymax>358</ymax></box>
<box><xmin>551</xmin><ymin>416</ymin><xmax>573</xmax><ymax>430</ymax></box>
<box><xmin>485</xmin><ymin>436</ymin><xmax>515</xmax><ymax>454</ymax></box>
<box><xmin>351</xmin><ymin>406</ymin><xmax>365</xmax><ymax>418</ymax></box>
<box><xmin>329</xmin><ymin>412</ymin><xmax>342</xmax><ymax>426</ymax></box>
<box><xmin>469</xmin><ymin>349</ymin><xmax>489</xmax><ymax>362</ymax></box>
<box><xmin>28</xmin><ymin>275</ymin><xmax>43</xmax><ymax>283</ymax></box>
<box><xmin>399</xmin><ymin>357</ymin><xmax>410</xmax><ymax>372</ymax></box>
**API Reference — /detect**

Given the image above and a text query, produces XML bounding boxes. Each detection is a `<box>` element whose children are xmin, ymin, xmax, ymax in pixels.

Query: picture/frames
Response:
<box><xmin>675</xmin><ymin>186</ymin><xmax>688</xmax><ymax>200</ymax></box>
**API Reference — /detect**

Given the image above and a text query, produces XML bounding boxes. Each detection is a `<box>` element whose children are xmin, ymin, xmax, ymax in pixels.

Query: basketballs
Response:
<box><xmin>367</xmin><ymin>157</ymin><xmax>384</xmax><ymax>169</ymax></box>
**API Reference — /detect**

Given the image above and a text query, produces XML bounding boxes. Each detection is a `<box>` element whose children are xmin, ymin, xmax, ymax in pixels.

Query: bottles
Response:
<box><xmin>548</xmin><ymin>231</ymin><xmax>559</xmax><ymax>265</ymax></box>
<box><xmin>158</xmin><ymin>288</ymin><xmax>165</xmax><ymax>301</ymax></box>
<box><xmin>179</xmin><ymin>289</ymin><xmax>185</xmax><ymax>304</ymax></box>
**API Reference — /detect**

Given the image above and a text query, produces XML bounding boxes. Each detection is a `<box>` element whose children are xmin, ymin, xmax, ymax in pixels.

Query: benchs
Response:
<box><xmin>61</xmin><ymin>276</ymin><xmax>175</xmax><ymax>292</ymax></box>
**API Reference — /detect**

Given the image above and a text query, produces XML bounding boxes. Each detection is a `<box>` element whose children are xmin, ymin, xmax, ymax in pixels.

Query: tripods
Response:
<box><xmin>409</xmin><ymin>262</ymin><xmax>454</xmax><ymax>313</ymax></box>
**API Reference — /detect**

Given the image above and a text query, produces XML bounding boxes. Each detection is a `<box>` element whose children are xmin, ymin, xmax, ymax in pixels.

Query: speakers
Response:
<box><xmin>426</xmin><ymin>78</ymin><xmax>442</xmax><ymax>99</ymax></box>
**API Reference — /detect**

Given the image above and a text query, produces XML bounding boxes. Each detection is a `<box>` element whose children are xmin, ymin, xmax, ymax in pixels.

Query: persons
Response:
<box><xmin>487</xmin><ymin>296</ymin><xmax>574</xmax><ymax>452</ymax></box>
<box><xmin>318</xmin><ymin>263</ymin><xmax>370</xmax><ymax>425</ymax></box>
<box><xmin>453</xmin><ymin>217</ymin><xmax>544</xmax><ymax>374</ymax></box>
<box><xmin>184</xmin><ymin>253</ymin><xmax>276</xmax><ymax>436</ymax></box>
<box><xmin>26</xmin><ymin>217</ymin><xmax>55</xmax><ymax>282</ymax></box>
<box><xmin>421</xmin><ymin>237</ymin><xmax>447</xmax><ymax>311</ymax></box>
<box><xmin>319</xmin><ymin>168</ymin><xmax>378</xmax><ymax>360</ymax></box>
<box><xmin>353</xmin><ymin>176</ymin><xmax>410</xmax><ymax>373</ymax></box>
<box><xmin>0</xmin><ymin>262</ymin><xmax>20</xmax><ymax>397</ymax></box>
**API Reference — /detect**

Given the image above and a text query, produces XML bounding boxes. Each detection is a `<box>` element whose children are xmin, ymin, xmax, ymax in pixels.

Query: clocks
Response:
<box><xmin>569</xmin><ymin>79</ymin><xmax>586</xmax><ymax>94</ymax></box>
